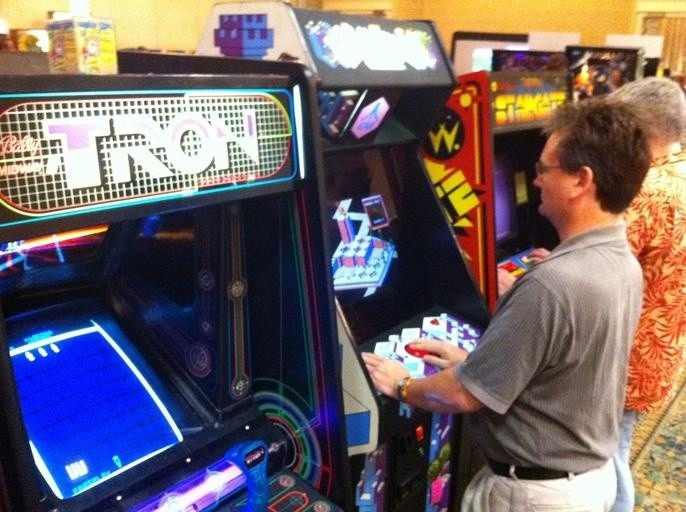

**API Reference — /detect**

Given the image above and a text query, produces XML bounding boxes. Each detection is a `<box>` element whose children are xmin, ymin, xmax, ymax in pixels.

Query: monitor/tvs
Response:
<box><xmin>492</xmin><ymin>152</ymin><xmax>518</xmax><ymax>243</ymax></box>
<box><xmin>9</xmin><ymin>300</ymin><xmax>206</xmax><ymax>500</ymax></box>
<box><xmin>322</xmin><ymin>149</ymin><xmax>435</xmax><ymax>344</ymax></box>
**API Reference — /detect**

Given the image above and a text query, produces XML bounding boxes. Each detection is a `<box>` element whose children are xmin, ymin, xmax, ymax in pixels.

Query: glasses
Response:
<box><xmin>536</xmin><ymin>162</ymin><xmax>581</xmax><ymax>174</ymax></box>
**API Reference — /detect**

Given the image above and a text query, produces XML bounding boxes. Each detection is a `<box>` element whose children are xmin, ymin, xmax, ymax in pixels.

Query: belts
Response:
<box><xmin>488</xmin><ymin>455</ymin><xmax>568</xmax><ymax>480</ymax></box>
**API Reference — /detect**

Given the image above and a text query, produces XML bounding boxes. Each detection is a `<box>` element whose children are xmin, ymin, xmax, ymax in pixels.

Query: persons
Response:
<box><xmin>363</xmin><ymin>99</ymin><xmax>654</xmax><ymax>512</ymax></box>
<box><xmin>497</xmin><ymin>76</ymin><xmax>686</xmax><ymax>512</ymax></box>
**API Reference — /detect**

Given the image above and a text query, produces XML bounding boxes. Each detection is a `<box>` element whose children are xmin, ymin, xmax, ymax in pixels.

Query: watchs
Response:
<box><xmin>397</xmin><ymin>377</ymin><xmax>410</xmax><ymax>402</ymax></box>
